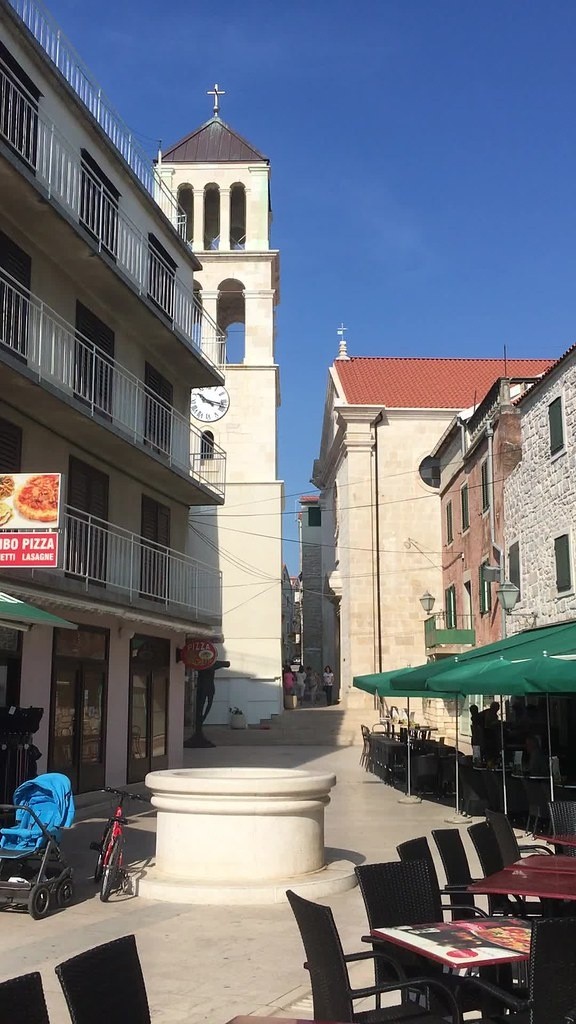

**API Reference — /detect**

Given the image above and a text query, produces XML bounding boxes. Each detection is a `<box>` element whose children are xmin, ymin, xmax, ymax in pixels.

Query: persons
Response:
<box><xmin>306</xmin><ymin>666</ymin><xmax>320</xmax><ymax>706</ymax></box>
<box><xmin>322</xmin><ymin>666</ymin><xmax>334</xmax><ymax>706</ymax></box>
<box><xmin>294</xmin><ymin>666</ymin><xmax>307</xmax><ymax>706</ymax></box>
<box><xmin>283</xmin><ymin>667</ymin><xmax>296</xmax><ymax>695</ymax></box>
<box><xmin>468</xmin><ymin>701</ymin><xmax>549</xmax><ymax>777</ymax></box>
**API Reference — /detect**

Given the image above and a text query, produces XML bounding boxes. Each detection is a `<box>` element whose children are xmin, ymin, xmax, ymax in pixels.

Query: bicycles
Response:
<box><xmin>88</xmin><ymin>783</ymin><xmax>153</xmax><ymax>902</ymax></box>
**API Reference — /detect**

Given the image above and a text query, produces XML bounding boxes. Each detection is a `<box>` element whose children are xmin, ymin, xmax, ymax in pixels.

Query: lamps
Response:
<box><xmin>495</xmin><ymin>581</ymin><xmax>519</xmax><ymax>613</ymax></box>
<box><xmin>420</xmin><ymin>589</ymin><xmax>435</xmax><ymax>615</ymax></box>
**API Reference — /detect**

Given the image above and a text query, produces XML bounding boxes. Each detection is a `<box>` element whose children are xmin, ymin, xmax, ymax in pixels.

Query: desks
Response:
<box><xmin>370</xmin><ymin>916</ymin><xmax>533</xmax><ymax>969</ymax></box>
<box><xmin>503</xmin><ymin>854</ymin><xmax>576</xmax><ymax>872</ymax></box>
<box><xmin>465</xmin><ymin>869</ymin><xmax>576</xmax><ymax>901</ymax></box>
<box><xmin>533</xmin><ymin>834</ymin><xmax>576</xmax><ymax>848</ymax></box>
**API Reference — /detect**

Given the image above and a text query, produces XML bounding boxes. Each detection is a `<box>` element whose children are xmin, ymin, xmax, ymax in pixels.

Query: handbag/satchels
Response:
<box><xmin>316</xmin><ymin>689</ymin><xmax>321</xmax><ymax>701</ymax></box>
<box><xmin>322</xmin><ymin>682</ymin><xmax>328</xmax><ymax>691</ymax></box>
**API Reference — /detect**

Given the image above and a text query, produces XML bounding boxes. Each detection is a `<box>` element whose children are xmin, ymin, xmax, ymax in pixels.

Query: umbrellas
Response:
<box><xmin>352</xmin><ymin>666</ymin><xmax>466</xmax><ymax>796</ymax></box>
<box><xmin>425</xmin><ymin>656</ymin><xmax>512</xmax><ymax>815</ymax></box>
<box><xmin>462</xmin><ymin>654</ymin><xmax>576</xmax><ymax>801</ymax></box>
<box><xmin>390</xmin><ymin>660</ymin><xmax>468</xmax><ymax>815</ymax></box>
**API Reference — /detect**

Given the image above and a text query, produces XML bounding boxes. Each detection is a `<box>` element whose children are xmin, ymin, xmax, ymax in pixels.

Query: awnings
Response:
<box><xmin>438</xmin><ymin>622</ymin><xmax>576</xmax><ymax>660</ymax></box>
<box><xmin>0</xmin><ymin>591</ymin><xmax>79</xmax><ymax>631</ymax></box>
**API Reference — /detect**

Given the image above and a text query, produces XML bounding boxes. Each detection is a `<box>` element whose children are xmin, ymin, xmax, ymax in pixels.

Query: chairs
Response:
<box><xmin>0</xmin><ymin>971</ymin><xmax>50</xmax><ymax>1024</ymax></box>
<box><xmin>354</xmin><ymin>858</ymin><xmax>500</xmax><ymax>1024</ymax></box>
<box><xmin>485</xmin><ymin>808</ymin><xmax>555</xmax><ymax>866</ymax></box>
<box><xmin>431</xmin><ymin>828</ymin><xmax>490</xmax><ymax>919</ymax></box>
<box><xmin>431</xmin><ymin>828</ymin><xmax>526</xmax><ymax>922</ymax></box>
<box><xmin>286</xmin><ymin>890</ymin><xmax>464</xmax><ymax>1024</ymax></box>
<box><xmin>54</xmin><ymin>934</ymin><xmax>155</xmax><ymax>1024</ymax></box>
<box><xmin>547</xmin><ymin>800</ymin><xmax>576</xmax><ymax>858</ymax></box>
<box><xmin>396</xmin><ymin>836</ymin><xmax>489</xmax><ymax>922</ymax></box>
<box><xmin>453</xmin><ymin>916</ymin><xmax>576</xmax><ymax>1024</ymax></box>
<box><xmin>359</xmin><ymin>723</ymin><xmax>576</xmax><ymax>836</ymax></box>
<box><xmin>468</xmin><ymin>821</ymin><xmax>544</xmax><ymax>919</ymax></box>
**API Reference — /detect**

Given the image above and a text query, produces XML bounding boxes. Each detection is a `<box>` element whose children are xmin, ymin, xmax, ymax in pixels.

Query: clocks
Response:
<box><xmin>189</xmin><ymin>386</ymin><xmax>230</xmax><ymax>423</ymax></box>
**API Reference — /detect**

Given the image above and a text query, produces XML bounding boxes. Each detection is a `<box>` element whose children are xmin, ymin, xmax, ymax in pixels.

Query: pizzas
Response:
<box><xmin>13</xmin><ymin>474</ymin><xmax>59</xmax><ymax>522</ymax></box>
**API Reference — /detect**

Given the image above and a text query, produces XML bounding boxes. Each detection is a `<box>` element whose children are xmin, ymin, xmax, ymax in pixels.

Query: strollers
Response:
<box><xmin>0</xmin><ymin>772</ymin><xmax>76</xmax><ymax>921</ymax></box>
